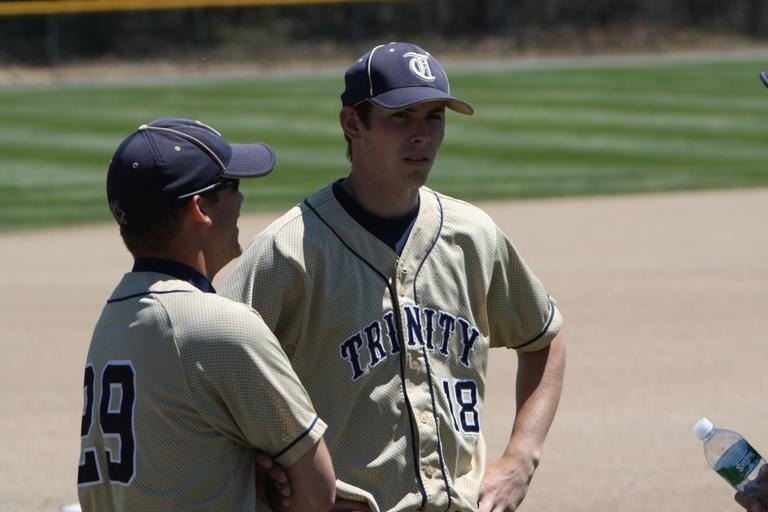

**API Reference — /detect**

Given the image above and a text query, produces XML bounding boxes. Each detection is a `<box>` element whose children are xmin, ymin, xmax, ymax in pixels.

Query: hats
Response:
<box><xmin>106</xmin><ymin>117</ymin><xmax>277</xmax><ymax>231</ymax></box>
<box><xmin>340</xmin><ymin>41</ymin><xmax>476</xmax><ymax>117</ymax></box>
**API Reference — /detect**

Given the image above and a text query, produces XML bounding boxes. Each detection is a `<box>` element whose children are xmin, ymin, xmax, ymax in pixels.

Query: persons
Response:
<box><xmin>734</xmin><ymin>461</ymin><xmax>768</xmax><ymax>512</ymax></box>
<box><xmin>220</xmin><ymin>41</ymin><xmax>566</xmax><ymax>512</ymax></box>
<box><xmin>76</xmin><ymin>115</ymin><xmax>337</xmax><ymax>512</ymax></box>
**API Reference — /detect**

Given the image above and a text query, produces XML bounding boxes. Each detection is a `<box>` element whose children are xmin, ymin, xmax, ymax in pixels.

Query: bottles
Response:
<box><xmin>691</xmin><ymin>416</ymin><xmax>768</xmax><ymax>499</ymax></box>
